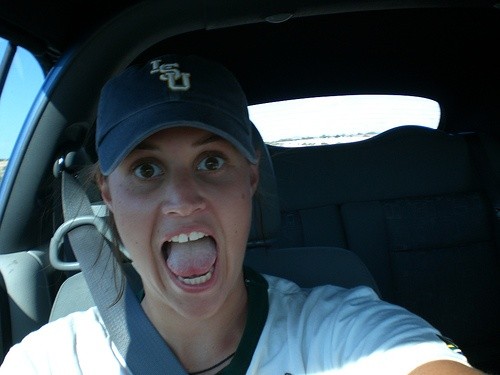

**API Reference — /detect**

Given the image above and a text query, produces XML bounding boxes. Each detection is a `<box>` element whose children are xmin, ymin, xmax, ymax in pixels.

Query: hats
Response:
<box><xmin>97</xmin><ymin>52</ymin><xmax>258</xmax><ymax>176</ymax></box>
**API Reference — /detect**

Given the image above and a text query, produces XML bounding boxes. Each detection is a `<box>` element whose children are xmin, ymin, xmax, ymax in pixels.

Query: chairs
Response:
<box><xmin>47</xmin><ymin>119</ymin><xmax>379</xmax><ymax>323</ymax></box>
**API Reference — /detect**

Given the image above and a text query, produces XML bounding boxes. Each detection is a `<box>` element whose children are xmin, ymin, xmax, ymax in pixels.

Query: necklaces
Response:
<box><xmin>187</xmin><ymin>352</ymin><xmax>235</xmax><ymax>375</ymax></box>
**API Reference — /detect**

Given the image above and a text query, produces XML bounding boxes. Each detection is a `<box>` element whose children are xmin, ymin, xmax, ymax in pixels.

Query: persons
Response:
<box><xmin>0</xmin><ymin>56</ymin><xmax>485</xmax><ymax>375</ymax></box>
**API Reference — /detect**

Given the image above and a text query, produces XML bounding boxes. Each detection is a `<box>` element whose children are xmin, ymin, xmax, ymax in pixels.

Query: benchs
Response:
<box><xmin>267</xmin><ymin>124</ymin><xmax>499</xmax><ymax>375</ymax></box>
